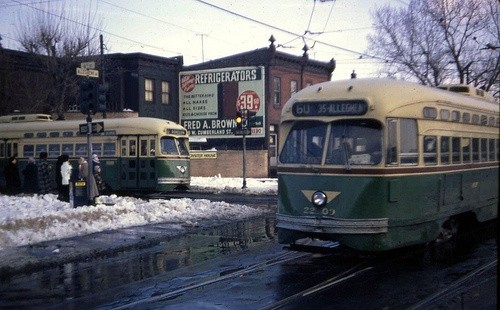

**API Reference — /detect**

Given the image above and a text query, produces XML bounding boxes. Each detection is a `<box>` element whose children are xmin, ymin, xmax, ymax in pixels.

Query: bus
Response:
<box><xmin>276</xmin><ymin>75</ymin><xmax>500</xmax><ymax>266</ymax></box>
<box><xmin>0</xmin><ymin>113</ymin><xmax>193</xmax><ymax>193</ymax></box>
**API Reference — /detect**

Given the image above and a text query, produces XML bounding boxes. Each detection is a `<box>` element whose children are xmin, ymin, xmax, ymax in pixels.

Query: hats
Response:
<box><xmin>8</xmin><ymin>156</ymin><xmax>16</xmax><ymax>162</ymax></box>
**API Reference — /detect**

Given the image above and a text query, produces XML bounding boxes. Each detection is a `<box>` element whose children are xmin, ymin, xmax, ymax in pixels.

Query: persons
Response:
<box><xmin>61</xmin><ymin>155</ymin><xmax>73</xmax><ymax>203</ymax></box>
<box><xmin>37</xmin><ymin>152</ymin><xmax>53</xmax><ymax>195</ymax></box>
<box><xmin>78</xmin><ymin>156</ymin><xmax>88</xmax><ymax>180</ymax></box>
<box><xmin>56</xmin><ymin>154</ymin><xmax>66</xmax><ymax>199</ymax></box>
<box><xmin>93</xmin><ymin>154</ymin><xmax>101</xmax><ymax>176</ymax></box>
<box><xmin>5</xmin><ymin>156</ymin><xmax>21</xmax><ymax>196</ymax></box>
<box><xmin>95</xmin><ymin>165</ymin><xmax>107</xmax><ymax>194</ymax></box>
<box><xmin>22</xmin><ymin>158</ymin><xmax>39</xmax><ymax>194</ymax></box>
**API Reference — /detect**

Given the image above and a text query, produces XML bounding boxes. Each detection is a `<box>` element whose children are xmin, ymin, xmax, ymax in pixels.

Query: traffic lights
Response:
<box><xmin>95</xmin><ymin>80</ymin><xmax>108</xmax><ymax>114</ymax></box>
<box><xmin>236</xmin><ymin>111</ymin><xmax>242</xmax><ymax>129</ymax></box>
<box><xmin>80</xmin><ymin>80</ymin><xmax>92</xmax><ymax>115</ymax></box>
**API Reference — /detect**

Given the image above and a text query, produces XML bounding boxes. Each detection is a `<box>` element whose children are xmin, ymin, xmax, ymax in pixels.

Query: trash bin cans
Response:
<box><xmin>271</xmin><ymin>169</ymin><xmax>277</xmax><ymax>178</ymax></box>
<box><xmin>69</xmin><ymin>180</ymin><xmax>87</xmax><ymax>209</ymax></box>
